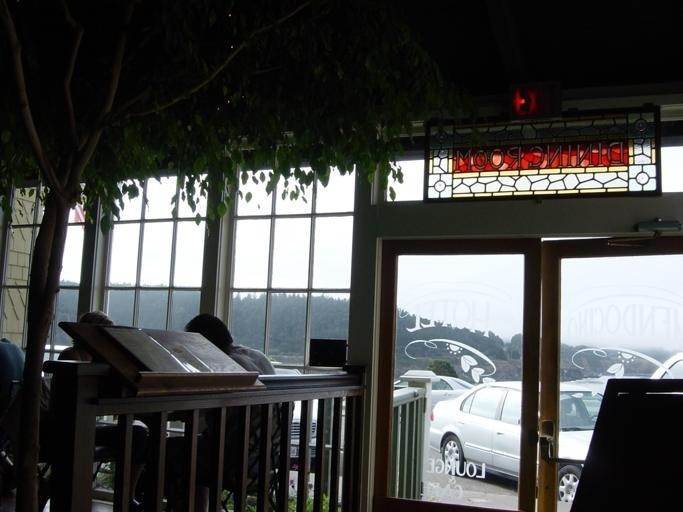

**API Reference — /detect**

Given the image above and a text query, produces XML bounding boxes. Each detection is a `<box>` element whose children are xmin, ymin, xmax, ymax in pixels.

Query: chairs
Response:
<box><xmin>186</xmin><ymin>398</ymin><xmax>288</xmax><ymax>512</ymax></box>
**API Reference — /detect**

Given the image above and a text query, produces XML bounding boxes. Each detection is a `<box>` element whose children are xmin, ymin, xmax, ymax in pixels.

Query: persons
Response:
<box><xmin>183</xmin><ymin>313</ymin><xmax>281</xmax><ymax>512</ymax></box>
<box><xmin>0</xmin><ymin>336</ymin><xmax>25</xmax><ymax>492</ymax></box>
<box><xmin>46</xmin><ymin>308</ymin><xmax>152</xmax><ymax>511</ymax></box>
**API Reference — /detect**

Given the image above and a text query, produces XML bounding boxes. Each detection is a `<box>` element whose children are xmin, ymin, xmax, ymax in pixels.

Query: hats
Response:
<box><xmin>82</xmin><ymin>311</ymin><xmax>113</xmax><ymax>325</ymax></box>
